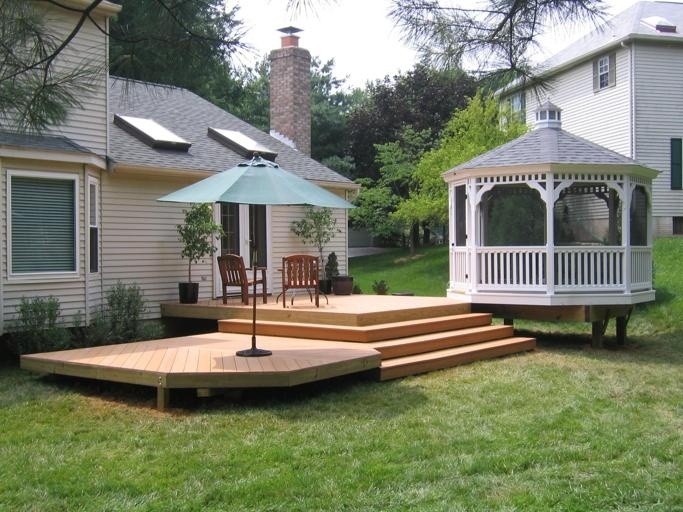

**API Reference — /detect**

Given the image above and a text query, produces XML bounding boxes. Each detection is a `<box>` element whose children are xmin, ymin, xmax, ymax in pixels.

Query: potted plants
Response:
<box><xmin>175</xmin><ymin>201</ymin><xmax>229</xmax><ymax>304</ymax></box>
<box><xmin>288</xmin><ymin>200</ymin><xmax>343</xmax><ymax>295</ymax></box>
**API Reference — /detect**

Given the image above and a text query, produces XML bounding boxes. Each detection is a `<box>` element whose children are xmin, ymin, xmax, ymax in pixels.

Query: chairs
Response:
<box><xmin>216</xmin><ymin>253</ymin><xmax>268</xmax><ymax>305</ymax></box>
<box><xmin>276</xmin><ymin>254</ymin><xmax>329</xmax><ymax>309</ymax></box>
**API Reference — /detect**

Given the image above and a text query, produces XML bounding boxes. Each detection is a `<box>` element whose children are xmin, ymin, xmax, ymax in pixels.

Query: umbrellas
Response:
<box><xmin>154</xmin><ymin>155</ymin><xmax>356</xmax><ymax>356</ymax></box>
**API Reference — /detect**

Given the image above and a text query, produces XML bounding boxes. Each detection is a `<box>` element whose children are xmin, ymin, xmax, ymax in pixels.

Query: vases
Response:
<box><xmin>332</xmin><ymin>276</ymin><xmax>354</xmax><ymax>296</ymax></box>
